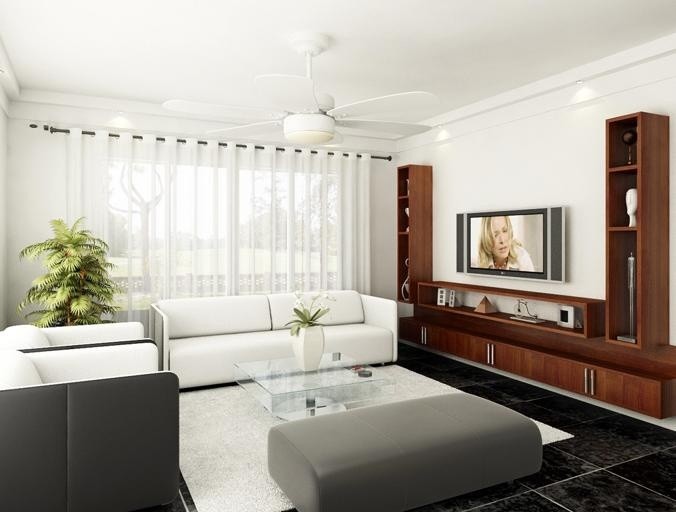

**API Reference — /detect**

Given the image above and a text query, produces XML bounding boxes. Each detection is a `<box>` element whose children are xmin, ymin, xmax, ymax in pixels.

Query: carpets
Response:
<box><xmin>179</xmin><ymin>362</ymin><xmax>574</xmax><ymax>512</ymax></box>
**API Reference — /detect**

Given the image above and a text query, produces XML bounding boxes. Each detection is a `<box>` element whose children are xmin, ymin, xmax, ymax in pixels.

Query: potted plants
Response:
<box><xmin>284</xmin><ymin>288</ymin><xmax>337</xmax><ymax>372</ymax></box>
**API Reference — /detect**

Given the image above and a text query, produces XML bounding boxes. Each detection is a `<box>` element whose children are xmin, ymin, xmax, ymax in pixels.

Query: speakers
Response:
<box><xmin>454</xmin><ymin>213</ymin><xmax>466</xmax><ymax>276</ymax></box>
<box><xmin>549</xmin><ymin>206</ymin><xmax>567</xmax><ymax>286</ymax></box>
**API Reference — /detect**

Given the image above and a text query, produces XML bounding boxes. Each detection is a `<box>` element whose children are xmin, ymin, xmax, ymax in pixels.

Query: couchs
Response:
<box><xmin>0</xmin><ymin>310</ymin><xmax>158</xmax><ymax>349</ymax></box>
<box><xmin>152</xmin><ymin>290</ymin><xmax>400</xmax><ymax>389</ymax></box>
<box><xmin>1</xmin><ymin>342</ymin><xmax>180</xmax><ymax>511</ymax></box>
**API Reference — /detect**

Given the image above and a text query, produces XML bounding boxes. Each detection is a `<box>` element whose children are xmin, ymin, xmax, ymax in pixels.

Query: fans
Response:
<box><xmin>160</xmin><ymin>32</ymin><xmax>439</xmax><ymax>147</ymax></box>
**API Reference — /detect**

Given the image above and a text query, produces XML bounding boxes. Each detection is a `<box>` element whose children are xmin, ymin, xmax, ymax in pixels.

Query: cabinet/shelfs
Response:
<box><xmin>415</xmin><ymin>280</ymin><xmax>675</xmax><ymax>420</ymax></box>
<box><xmin>396</xmin><ymin>163</ymin><xmax>433</xmax><ymax>303</ymax></box>
<box><xmin>606</xmin><ymin>111</ymin><xmax>671</xmax><ymax>349</ymax></box>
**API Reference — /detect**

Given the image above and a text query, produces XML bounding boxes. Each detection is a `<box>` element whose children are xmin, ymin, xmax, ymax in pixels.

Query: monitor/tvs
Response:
<box><xmin>467</xmin><ymin>207</ymin><xmax>548</xmax><ymax>281</ymax></box>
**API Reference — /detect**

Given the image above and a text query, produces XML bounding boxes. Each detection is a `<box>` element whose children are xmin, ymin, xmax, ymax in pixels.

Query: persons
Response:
<box><xmin>477</xmin><ymin>215</ymin><xmax>535</xmax><ymax>272</ymax></box>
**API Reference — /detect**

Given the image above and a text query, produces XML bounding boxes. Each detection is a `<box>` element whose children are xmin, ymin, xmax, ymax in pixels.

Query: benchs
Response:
<box><xmin>268</xmin><ymin>394</ymin><xmax>543</xmax><ymax>512</ymax></box>
